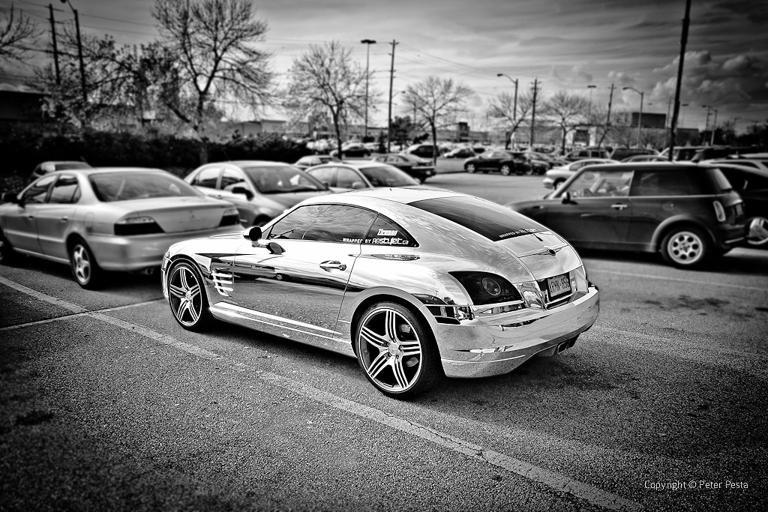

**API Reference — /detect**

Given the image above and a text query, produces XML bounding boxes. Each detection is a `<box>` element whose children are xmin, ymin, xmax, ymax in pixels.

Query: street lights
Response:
<box><xmin>623</xmin><ymin>85</ymin><xmax>645</xmax><ymax>148</ymax></box>
<box><xmin>665</xmin><ymin>96</ymin><xmax>688</xmax><ymax>126</ymax></box>
<box><xmin>699</xmin><ymin>105</ymin><xmax>719</xmax><ymax>146</ymax></box>
<box><xmin>494</xmin><ymin>73</ymin><xmax>518</xmax><ymax>131</ymax></box>
<box><xmin>359</xmin><ymin>35</ymin><xmax>377</xmax><ymax>138</ymax></box>
<box><xmin>587</xmin><ymin>85</ymin><xmax>617</xmax><ymax>144</ymax></box>
